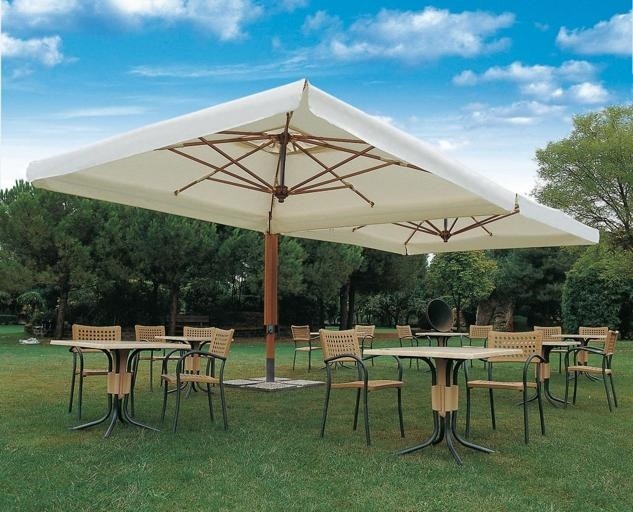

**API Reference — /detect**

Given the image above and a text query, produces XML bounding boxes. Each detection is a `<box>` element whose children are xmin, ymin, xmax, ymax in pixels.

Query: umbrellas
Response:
<box><xmin>26</xmin><ymin>78</ymin><xmax>601</xmax><ymax>391</ymax></box>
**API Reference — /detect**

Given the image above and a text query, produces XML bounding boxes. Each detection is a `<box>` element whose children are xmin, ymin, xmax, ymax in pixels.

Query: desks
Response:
<box><xmin>362</xmin><ymin>346</ymin><xmax>523</xmax><ymax>466</ymax></box>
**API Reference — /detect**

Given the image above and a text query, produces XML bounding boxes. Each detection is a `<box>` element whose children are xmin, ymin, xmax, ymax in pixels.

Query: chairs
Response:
<box><xmin>292</xmin><ymin>325</ymin><xmax>376</xmax><ymax>371</ymax></box>
<box><xmin>68</xmin><ymin>324</ymin><xmax>234</xmax><ymax>434</ymax></box>
<box><xmin>397</xmin><ymin>325</ymin><xmax>495</xmax><ymax>373</ymax></box>
<box><xmin>564</xmin><ymin>330</ymin><xmax>618</xmax><ymax>412</ymax></box>
<box><xmin>515</xmin><ymin>326</ymin><xmax>610</xmax><ymax>410</ymax></box>
<box><xmin>466</xmin><ymin>330</ymin><xmax>547</xmax><ymax>444</ymax></box>
<box><xmin>319</xmin><ymin>329</ymin><xmax>406</xmax><ymax>447</ymax></box>
